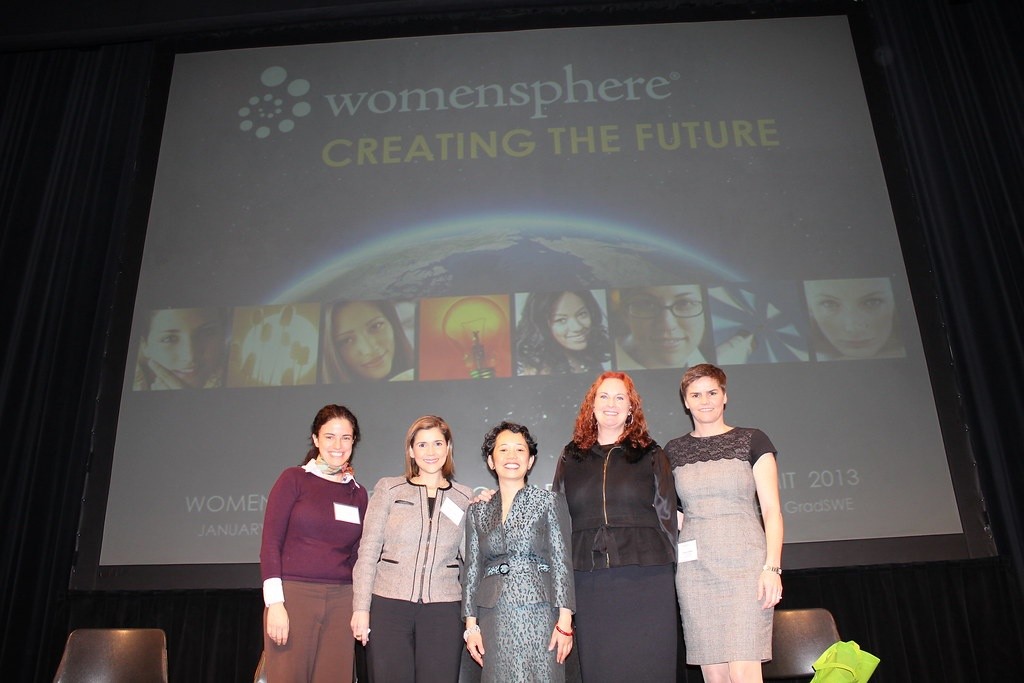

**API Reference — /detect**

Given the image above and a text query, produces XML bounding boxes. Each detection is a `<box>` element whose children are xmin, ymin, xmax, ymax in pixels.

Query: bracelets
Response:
<box><xmin>463</xmin><ymin>625</ymin><xmax>479</xmax><ymax>642</ymax></box>
<box><xmin>555</xmin><ymin>623</ymin><xmax>574</xmax><ymax>636</ymax></box>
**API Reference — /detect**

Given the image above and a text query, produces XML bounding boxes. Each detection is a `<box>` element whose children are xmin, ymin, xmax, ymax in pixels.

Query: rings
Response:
<box><xmin>356</xmin><ymin>635</ymin><xmax>362</xmax><ymax>637</ymax></box>
<box><xmin>777</xmin><ymin>597</ymin><xmax>782</xmax><ymax>599</ymax></box>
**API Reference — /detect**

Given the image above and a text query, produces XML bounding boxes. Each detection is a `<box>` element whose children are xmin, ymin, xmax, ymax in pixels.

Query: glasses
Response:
<box><xmin>618</xmin><ymin>299</ymin><xmax>705</xmax><ymax>318</ymax></box>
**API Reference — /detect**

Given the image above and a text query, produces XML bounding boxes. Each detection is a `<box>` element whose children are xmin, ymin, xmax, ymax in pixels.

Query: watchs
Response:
<box><xmin>763</xmin><ymin>566</ymin><xmax>782</xmax><ymax>574</ymax></box>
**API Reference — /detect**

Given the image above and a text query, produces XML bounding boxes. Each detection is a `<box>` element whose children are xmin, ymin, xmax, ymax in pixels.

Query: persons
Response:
<box><xmin>460</xmin><ymin>422</ymin><xmax>577</xmax><ymax>683</ymax></box>
<box><xmin>350</xmin><ymin>415</ymin><xmax>475</xmax><ymax>683</ymax></box>
<box><xmin>516</xmin><ymin>289</ymin><xmax>611</xmax><ymax>375</ymax></box>
<box><xmin>664</xmin><ymin>364</ymin><xmax>784</xmax><ymax>683</ymax></box>
<box><xmin>470</xmin><ymin>372</ymin><xmax>680</xmax><ymax>683</ymax></box>
<box><xmin>322</xmin><ymin>300</ymin><xmax>414</xmax><ymax>381</ymax></box>
<box><xmin>615</xmin><ymin>284</ymin><xmax>706</xmax><ymax>369</ymax></box>
<box><xmin>142</xmin><ymin>306</ymin><xmax>225</xmax><ymax>387</ymax></box>
<box><xmin>804</xmin><ymin>278</ymin><xmax>905</xmax><ymax>362</ymax></box>
<box><xmin>255</xmin><ymin>404</ymin><xmax>369</xmax><ymax>683</ymax></box>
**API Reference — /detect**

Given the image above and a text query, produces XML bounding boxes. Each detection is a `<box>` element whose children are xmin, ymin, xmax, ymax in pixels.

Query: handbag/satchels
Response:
<box><xmin>808</xmin><ymin>640</ymin><xmax>880</xmax><ymax>683</ymax></box>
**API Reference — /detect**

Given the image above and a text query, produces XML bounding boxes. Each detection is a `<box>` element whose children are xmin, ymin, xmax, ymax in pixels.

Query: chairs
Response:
<box><xmin>761</xmin><ymin>608</ymin><xmax>840</xmax><ymax>683</ymax></box>
<box><xmin>53</xmin><ymin>628</ymin><xmax>168</xmax><ymax>683</ymax></box>
